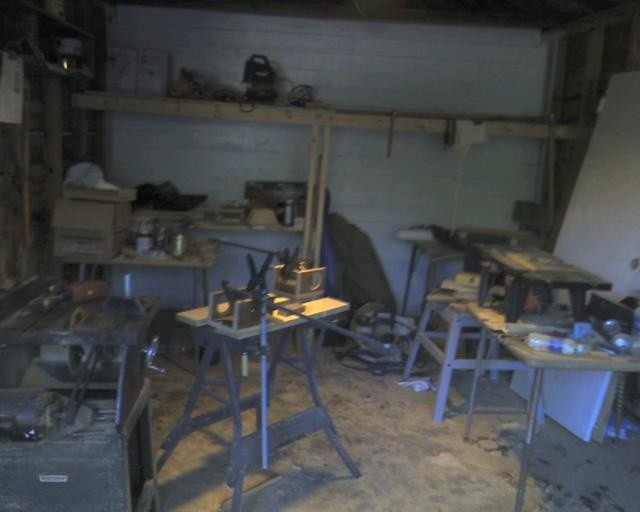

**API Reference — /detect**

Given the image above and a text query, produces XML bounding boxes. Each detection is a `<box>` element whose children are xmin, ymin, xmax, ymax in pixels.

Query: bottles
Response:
<box><xmin>631</xmin><ymin>304</ymin><xmax>639</xmax><ymax>353</ymax></box>
<box><xmin>239</xmin><ymin>352</ymin><xmax>248</xmax><ymax>377</ymax></box>
<box><xmin>284</xmin><ymin>199</ymin><xmax>292</xmax><ymax>224</ymax></box>
<box><xmin>527</xmin><ymin>333</ymin><xmax>590</xmax><ymax>355</ymax></box>
<box><xmin>135</xmin><ymin>230</ymin><xmax>156</xmax><ymax>253</ymax></box>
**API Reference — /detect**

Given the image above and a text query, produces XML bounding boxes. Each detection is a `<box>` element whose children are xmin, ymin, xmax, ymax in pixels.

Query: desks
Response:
<box><xmin>156</xmin><ymin>286</ymin><xmax>362</xmax><ymax>512</ymax></box>
<box><xmin>48</xmin><ymin>232</ymin><xmax>221</xmax><ymax>370</ymax></box>
<box><xmin>395</xmin><ymin>224</ymin><xmax>639</xmax><ymax>512</ymax></box>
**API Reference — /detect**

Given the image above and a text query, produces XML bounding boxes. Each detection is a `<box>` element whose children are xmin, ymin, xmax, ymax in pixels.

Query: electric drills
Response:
<box><xmin>43</xmin><ymin>278</ymin><xmax>108</xmax><ymax>304</ymax></box>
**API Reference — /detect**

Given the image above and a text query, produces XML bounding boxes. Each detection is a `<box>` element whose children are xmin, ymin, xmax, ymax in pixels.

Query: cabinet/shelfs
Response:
<box><xmin>1</xmin><ymin>293</ymin><xmax>155</xmax><ymax>512</ymax></box>
<box><xmin>1</xmin><ymin>0</ymin><xmax>111</xmax><ymax>292</ymax></box>
<box><xmin>76</xmin><ymin>87</ymin><xmax>594</xmax><ymax>359</ymax></box>
